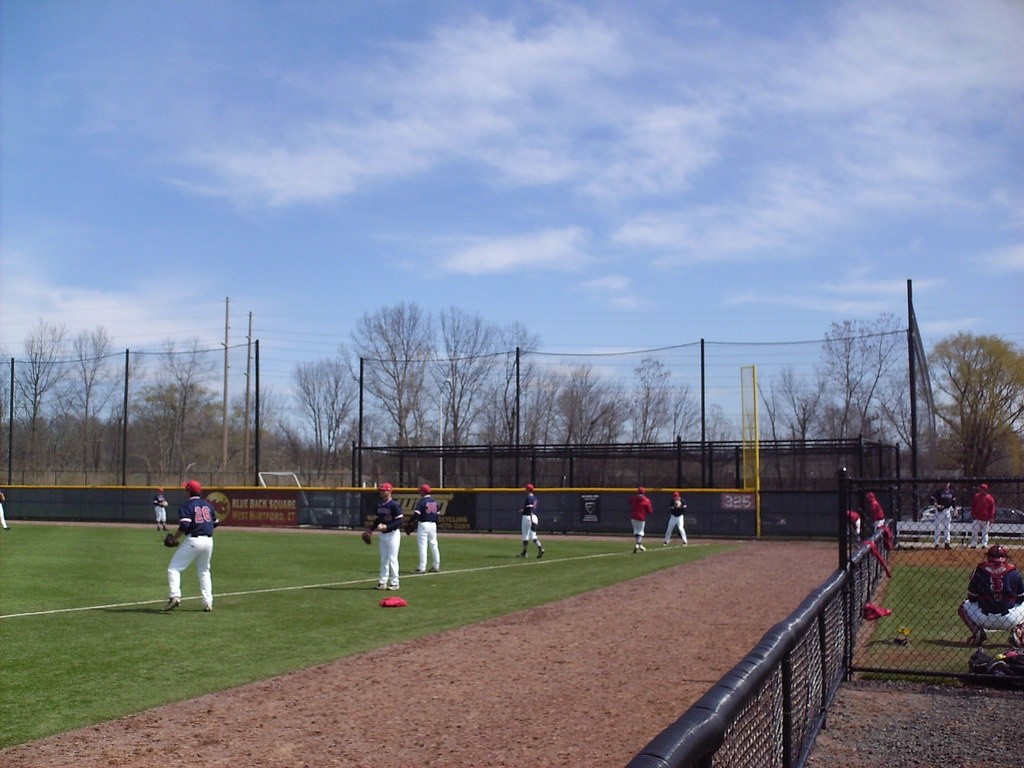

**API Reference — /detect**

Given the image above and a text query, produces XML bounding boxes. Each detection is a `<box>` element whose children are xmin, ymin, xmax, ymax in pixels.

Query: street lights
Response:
<box><xmin>439</xmin><ymin>379</ymin><xmax>452</xmax><ymax>488</ymax></box>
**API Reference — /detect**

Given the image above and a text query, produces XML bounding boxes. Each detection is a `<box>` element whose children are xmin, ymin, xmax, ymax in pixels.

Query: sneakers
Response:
<box><xmin>967</xmin><ymin>628</ymin><xmax>987</xmax><ymax>647</ymax></box>
<box><xmin>1007</xmin><ymin>625</ymin><xmax>1024</xmax><ymax>647</ymax></box>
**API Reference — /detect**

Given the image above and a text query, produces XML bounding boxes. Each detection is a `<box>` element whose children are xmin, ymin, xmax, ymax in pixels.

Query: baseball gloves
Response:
<box><xmin>164</xmin><ymin>533</ymin><xmax>180</xmax><ymax>548</ymax></box>
<box><xmin>937</xmin><ymin>505</ymin><xmax>945</xmax><ymax>512</ymax></box>
<box><xmin>531</xmin><ymin>522</ymin><xmax>537</xmax><ymax>531</ymax></box>
<box><xmin>405</xmin><ymin>523</ymin><xmax>415</xmax><ymax>535</ymax></box>
<box><xmin>362</xmin><ymin>530</ymin><xmax>372</xmax><ymax>544</ymax></box>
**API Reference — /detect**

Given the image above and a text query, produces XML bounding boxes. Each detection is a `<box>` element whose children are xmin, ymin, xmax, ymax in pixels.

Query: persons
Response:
<box><xmin>154</xmin><ymin>488</ymin><xmax>169</xmax><ymax>531</ymax></box>
<box><xmin>867</xmin><ymin>493</ymin><xmax>885</xmax><ymax>531</ymax></box>
<box><xmin>406</xmin><ymin>485</ymin><xmax>440</xmax><ymax>574</ymax></box>
<box><xmin>930</xmin><ymin>483</ymin><xmax>957</xmax><ymax>550</ymax></box>
<box><xmin>362</xmin><ymin>483</ymin><xmax>404</xmax><ymax>590</ymax></box>
<box><xmin>847</xmin><ymin>510</ymin><xmax>861</xmax><ymax>550</ymax></box>
<box><xmin>663</xmin><ymin>492</ymin><xmax>687</xmax><ymax>546</ymax></box>
<box><xmin>164</xmin><ymin>480</ymin><xmax>220</xmax><ymax>612</ymax></box>
<box><xmin>516</xmin><ymin>484</ymin><xmax>544</xmax><ymax>558</ymax></box>
<box><xmin>958</xmin><ymin>545</ymin><xmax>1024</xmax><ymax>647</ymax></box>
<box><xmin>970</xmin><ymin>484</ymin><xmax>995</xmax><ymax>548</ymax></box>
<box><xmin>628</xmin><ymin>488</ymin><xmax>652</xmax><ymax>552</ymax></box>
<box><xmin>0</xmin><ymin>492</ymin><xmax>10</xmax><ymax>530</ymax></box>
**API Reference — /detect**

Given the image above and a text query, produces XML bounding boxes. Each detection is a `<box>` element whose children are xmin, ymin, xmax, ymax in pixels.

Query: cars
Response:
<box><xmin>918</xmin><ymin>504</ymin><xmax>1024</xmax><ymax>525</ymax></box>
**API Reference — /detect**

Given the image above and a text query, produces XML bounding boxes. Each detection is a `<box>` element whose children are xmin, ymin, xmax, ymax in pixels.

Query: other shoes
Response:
<box><xmin>202</xmin><ymin>604</ymin><xmax>213</xmax><ymax>612</ymax></box>
<box><xmin>387</xmin><ymin>584</ymin><xmax>399</xmax><ymax>590</ymax></box>
<box><xmin>411</xmin><ymin>569</ymin><xmax>425</xmax><ymax>574</ymax></box>
<box><xmin>632</xmin><ymin>544</ymin><xmax>639</xmax><ymax>554</ymax></box>
<box><xmin>428</xmin><ymin>568</ymin><xmax>440</xmax><ymax>572</ymax></box>
<box><xmin>934</xmin><ymin>544</ymin><xmax>939</xmax><ymax>550</ymax></box>
<box><xmin>515</xmin><ymin>552</ymin><xmax>529</xmax><ymax>558</ymax></box>
<box><xmin>945</xmin><ymin>542</ymin><xmax>952</xmax><ymax>550</ymax></box>
<box><xmin>536</xmin><ymin>548</ymin><xmax>545</xmax><ymax>558</ymax></box>
<box><xmin>639</xmin><ymin>544</ymin><xmax>646</xmax><ymax>551</ymax></box>
<box><xmin>969</xmin><ymin>546</ymin><xmax>976</xmax><ymax>549</ymax></box>
<box><xmin>375</xmin><ymin>583</ymin><xmax>386</xmax><ymax>589</ymax></box>
<box><xmin>981</xmin><ymin>546</ymin><xmax>988</xmax><ymax>549</ymax></box>
<box><xmin>163</xmin><ymin>597</ymin><xmax>181</xmax><ymax>612</ymax></box>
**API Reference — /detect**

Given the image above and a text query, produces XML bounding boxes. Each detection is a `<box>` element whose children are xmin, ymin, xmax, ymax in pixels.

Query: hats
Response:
<box><xmin>523</xmin><ymin>484</ymin><xmax>533</xmax><ymax>490</ymax></box>
<box><xmin>638</xmin><ymin>487</ymin><xmax>646</xmax><ymax>493</ymax></box>
<box><xmin>157</xmin><ymin>487</ymin><xmax>164</xmax><ymax>493</ymax></box>
<box><xmin>180</xmin><ymin>480</ymin><xmax>202</xmax><ymax>493</ymax></box>
<box><xmin>672</xmin><ymin>491</ymin><xmax>680</xmax><ymax>498</ymax></box>
<box><xmin>420</xmin><ymin>484</ymin><xmax>432</xmax><ymax>493</ymax></box>
<box><xmin>976</xmin><ymin>483</ymin><xmax>988</xmax><ymax>490</ymax></box>
<box><xmin>376</xmin><ymin>483</ymin><xmax>392</xmax><ymax>490</ymax></box>
<box><xmin>986</xmin><ymin>544</ymin><xmax>1007</xmax><ymax>563</ymax></box>
